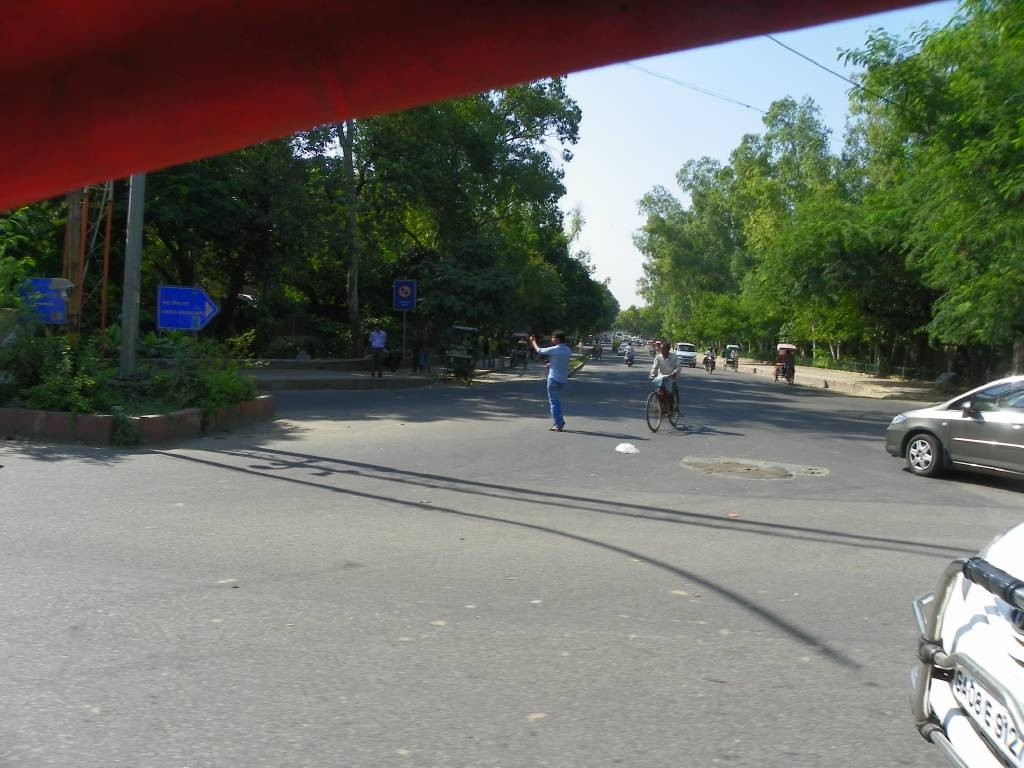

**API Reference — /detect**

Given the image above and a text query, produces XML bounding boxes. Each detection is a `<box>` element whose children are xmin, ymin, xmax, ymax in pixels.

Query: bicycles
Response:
<box><xmin>645</xmin><ymin>370</ymin><xmax>682</xmax><ymax>431</ymax></box>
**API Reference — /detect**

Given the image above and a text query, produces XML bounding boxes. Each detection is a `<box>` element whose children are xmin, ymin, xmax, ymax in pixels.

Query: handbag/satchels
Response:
<box><xmin>657</xmin><ymin>387</ymin><xmax>673</xmax><ymax>402</ymax></box>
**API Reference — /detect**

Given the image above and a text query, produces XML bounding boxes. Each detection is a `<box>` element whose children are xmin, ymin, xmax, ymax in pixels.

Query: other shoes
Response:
<box><xmin>551</xmin><ymin>425</ymin><xmax>563</xmax><ymax>431</ymax></box>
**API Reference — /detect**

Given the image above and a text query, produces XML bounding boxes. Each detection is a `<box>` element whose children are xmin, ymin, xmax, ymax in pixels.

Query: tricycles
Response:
<box><xmin>723</xmin><ymin>345</ymin><xmax>741</xmax><ymax>373</ymax></box>
<box><xmin>509</xmin><ymin>332</ymin><xmax>530</xmax><ymax>367</ymax></box>
<box><xmin>442</xmin><ymin>325</ymin><xmax>480</xmax><ymax>385</ymax></box>
<box><xmin>774</xmin><ymin>344</ymin><xmax>797</xmax><ymax>385</ymax></box>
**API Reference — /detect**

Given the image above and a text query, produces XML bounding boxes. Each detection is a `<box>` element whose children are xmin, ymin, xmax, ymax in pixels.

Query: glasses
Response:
<box><xmin>549</xmin><ymin>337</ymin><xmax>556</xmax><ymax>338</ymax></box>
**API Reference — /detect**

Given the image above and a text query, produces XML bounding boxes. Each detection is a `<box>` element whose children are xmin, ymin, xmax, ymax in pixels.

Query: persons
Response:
<box><xmin>489</xmin><ymin>335</ymin><xmax>498</xmax><ymax>369</ymax></box>
<box><xmin>650</xmin><ymin>343</ymin><xmax>681</xmax><ymax>415</ymax></box>
<box><xmin>781</xmin><ymin>350</ymin><xmax>793</xmax><ymax>373</ymax></box>
<box><xmin>704</xmin><ymin>347</ymin><xmax>714</xmax><ymax>359</ymax></box>
<box><xmin>369</xmin><ymin>324</ymin><xmax>386</xmax><ymax>377</ymax></box>
<box><xmin>650</xmin><ymin>343</ymin><xmax>655</xmax><ymax>351</ymax></box>
<box><xmin>623</xmin><ymin>342</ymin><xmax>634</xmax><ymax>355</ymax></box>
<box><xmin>730</xmin><ymin>348</ymin><xmax>738</xmax><ymax>359</ymax></box>
<box><xmin>413</xmin><ymin>328</ymin><xmax>420</xmax><ymax>372</ymax></box>
<box><xmin>419</xmin><ymin>327</ymin><xmax>431</xmax><ymax>370</ymax></box>
<box><xmin>593</xmin><ymin>340</ymin><xmax>601</xmax><ymax>351</ymax></box>
<box><xmin>529</xmin><ymin>330</ymin><xmax>572</xmax><ymax>432</ymax></box>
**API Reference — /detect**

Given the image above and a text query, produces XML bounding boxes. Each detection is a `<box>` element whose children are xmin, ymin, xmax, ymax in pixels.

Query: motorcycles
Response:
<box><xmin>703</xmin><ymin>354</ymin><xmax>718</xmax><ymax>374</ymax></box>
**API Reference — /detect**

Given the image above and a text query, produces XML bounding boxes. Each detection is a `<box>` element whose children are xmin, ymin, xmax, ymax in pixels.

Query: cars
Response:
<box><xmin>885</xmin><ymin>376</ymin><xmax>1024</xmax><ymax>478</ymax></box>
<box><xmin>590</xmin><ymin>330</ymin><xmax>665</xmax><ymax>367</ymax></box>
<box><xmin>674</xmin><ymin>341</ymin><xmax>698</xmax><ymax>368</ymax></box>
<box><xmin>910</xmin><ymin>518</ymin><xmax>1024</xmax><ymax>767</ymax></box>
